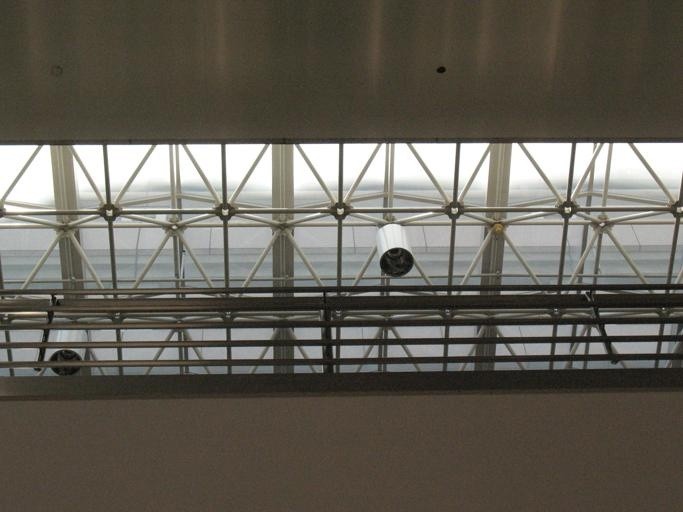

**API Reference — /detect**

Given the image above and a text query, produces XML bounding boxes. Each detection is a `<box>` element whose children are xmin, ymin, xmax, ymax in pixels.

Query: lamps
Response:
<box><xmin>46</xmin><ymin>328</ymin><xmax>88</xmax><ymax>377</ymax></box>
<box><xmin>374</xmin><ymin>223</ymin><xmax>414</xmax><ymax>278</ymax></box>
